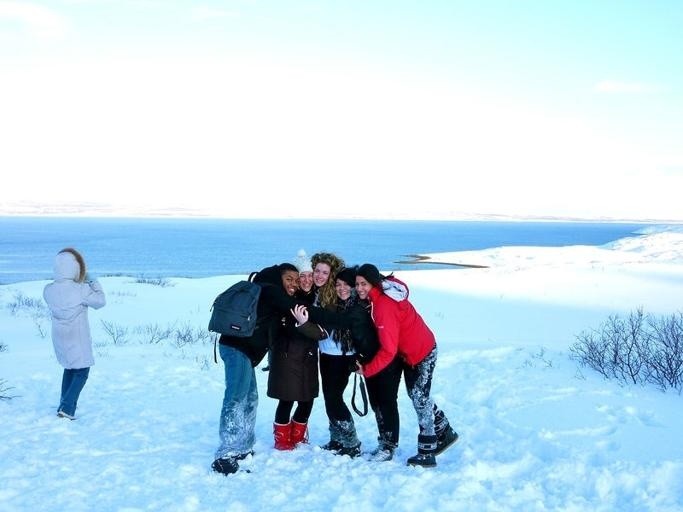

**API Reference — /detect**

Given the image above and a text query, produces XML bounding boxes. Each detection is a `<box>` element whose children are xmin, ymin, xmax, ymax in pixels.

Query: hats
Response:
<box><xmin>295</xmin><ymin>250</ymin><xmax>314</xmax><ymax>278</ymax></box>
<box><xmin>339</xmin><ymin>267</ymin><xmax>356</xmax><ymax>288</ymax></box>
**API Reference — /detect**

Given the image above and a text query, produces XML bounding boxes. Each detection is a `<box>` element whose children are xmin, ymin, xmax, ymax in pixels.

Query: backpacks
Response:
<box><xmin>208</xmin><ymin>272</ymin><xmax>277</xmax><ymax>337</ymax></box>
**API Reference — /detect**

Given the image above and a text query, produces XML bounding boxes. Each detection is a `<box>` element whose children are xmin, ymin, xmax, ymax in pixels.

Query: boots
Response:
<box><xmin>290</xmin><ymin>419</ymin><xmax>307</xmax><ymax>444</ymax></box>
<box><xmin>274</xmin><ymin>422</ymin><xmax>295</xmax><ymax>450</ymax></box>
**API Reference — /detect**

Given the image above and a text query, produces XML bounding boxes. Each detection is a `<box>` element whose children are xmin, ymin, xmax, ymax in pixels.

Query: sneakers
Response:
<box><xmin>320</xmin><ymin>441</ymin><xmax>343</xmax><ymax>451</ymax></box>
<box><xmin>433</xmin><ymin>427</ymin><xmax>459</xmax><ymax>455</ymax></box>
<box><xmin>58</xmin><ymin>410</ymin><xmax>76</xmax><ymax>420</ymax></box>
<box><xmin>372</xmin><ymin>442</ymin><xmax>395</xmax><ymax>461</ymax></box>
<box><xmin>211</xmin><ymin>457</ymin><xmax>252</xmax><ymax>475</ymax></box>
<box><xmin>338</xmin><ymin>442</ymin><xmax>361</xmax><ymax>457</ymax></box>
<box><xmin>407</xmin><ymin>453</ymin><xmax>437</xmax><ymax>467</ymax></box>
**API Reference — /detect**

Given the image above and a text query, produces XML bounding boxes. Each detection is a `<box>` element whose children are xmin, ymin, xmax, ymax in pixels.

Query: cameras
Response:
<box><xmin>349</xmin><ymin>356</ymin><xmax>359</xmax><ymax>372</ymax></box>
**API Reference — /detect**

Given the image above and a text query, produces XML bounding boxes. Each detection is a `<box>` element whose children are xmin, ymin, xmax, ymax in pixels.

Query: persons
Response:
<box><xmin>335</xmin><ymin>265</ymin><xmax>403</xmax><ymax>463</ymax></box>
<box><xmin>310</xmin><ymin>251</ymin><xmax>363</xmax><ymax>458</ymax></box>
<box><xmin>43</xmin><ymin>247</ymin><xmax>106</xmax><ymax>420</ymax></box>
<box><xmin>354</xmin><ymin>264</ymin><xmax>458</xmax><ymax>467</ymax></box>
<box><xmin>210</xmin><ymin>261</ymin><xmax>351</xmax><ymax>472</ymax></box>
<box><xmin>266</xmin><ymin>249</ymin><xmax>319</xmax><ymax>450</ymax></box>
